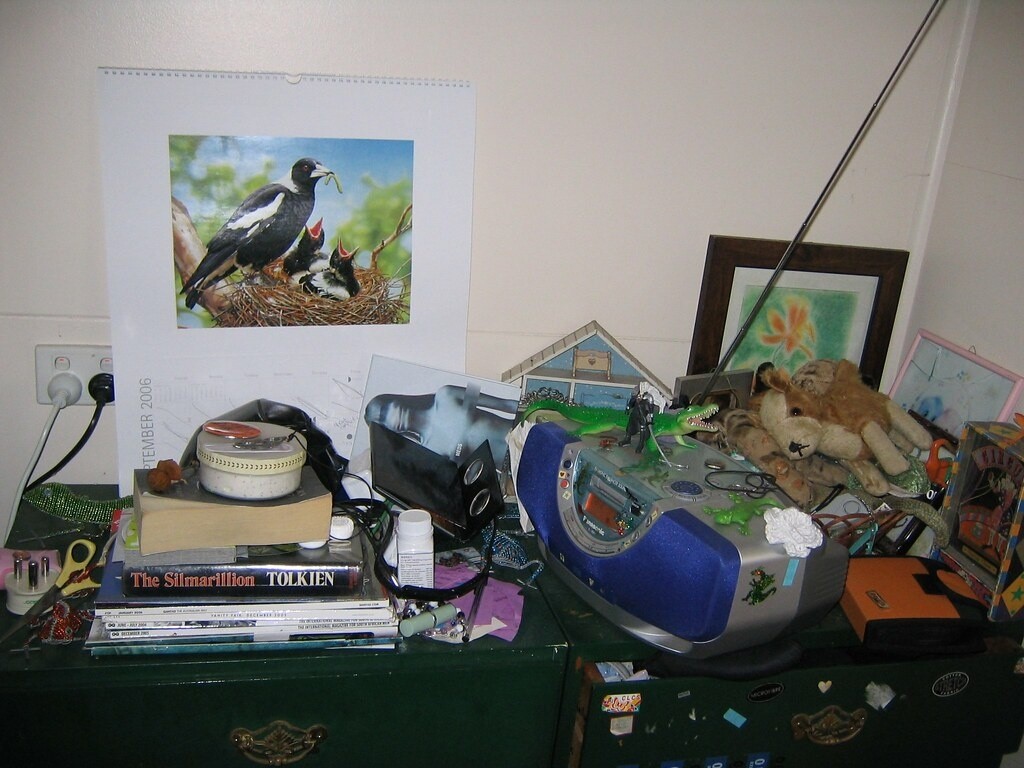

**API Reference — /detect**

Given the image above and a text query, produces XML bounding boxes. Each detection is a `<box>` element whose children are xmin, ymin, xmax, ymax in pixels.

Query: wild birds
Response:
<box><xmin>177</xmin><ymin>158</ymin><xmax>359</xmax><ymax>309</ymax></box>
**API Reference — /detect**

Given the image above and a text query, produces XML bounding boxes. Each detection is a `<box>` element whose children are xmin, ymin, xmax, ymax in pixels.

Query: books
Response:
<box><xmin>83</xmin><ymin>507</ymin><xmax>404</xmax><ymax>657</ymax></box>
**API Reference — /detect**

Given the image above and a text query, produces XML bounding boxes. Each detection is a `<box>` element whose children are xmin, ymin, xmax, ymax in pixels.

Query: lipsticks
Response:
<box><xmin>399</xmin><ymin>602</ymin><xmax>459</xmax><ymax>638</ymax></box>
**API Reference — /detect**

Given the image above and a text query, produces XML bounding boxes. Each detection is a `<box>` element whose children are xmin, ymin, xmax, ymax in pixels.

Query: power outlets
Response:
<box><xmin>34</xmin><ymin>344</ymin><xmax>116</xmax><ymax>407</ymax></box>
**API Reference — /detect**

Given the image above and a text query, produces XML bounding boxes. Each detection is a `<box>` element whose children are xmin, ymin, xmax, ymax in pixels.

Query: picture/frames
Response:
<box><xmin>887</xmin><ymin>328</ymin><xmax>1023</xmax><ymax>438</ymax></box>
<box><xmin>685</xmin><ymin>234</ymin><xmax>912</xmax><ymax>394</ymax></box>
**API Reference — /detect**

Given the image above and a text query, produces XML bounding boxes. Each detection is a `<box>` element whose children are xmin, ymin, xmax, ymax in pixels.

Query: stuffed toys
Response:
<box><xmin>712</xmin><ymin>355</ymin><xmax>932</xmax><ymax>512</ymax></box>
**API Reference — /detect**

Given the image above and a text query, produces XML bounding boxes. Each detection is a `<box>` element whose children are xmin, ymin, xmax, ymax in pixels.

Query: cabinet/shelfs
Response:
<box><xmin>0</xmin><ymin>484</ymin><xmax>567</xmax><ymax>768</ymax></box>
<box><xmin>515</xmin><ymin>530</ymin><xmax>1024</xmax><ymax>768</ymax></box>
